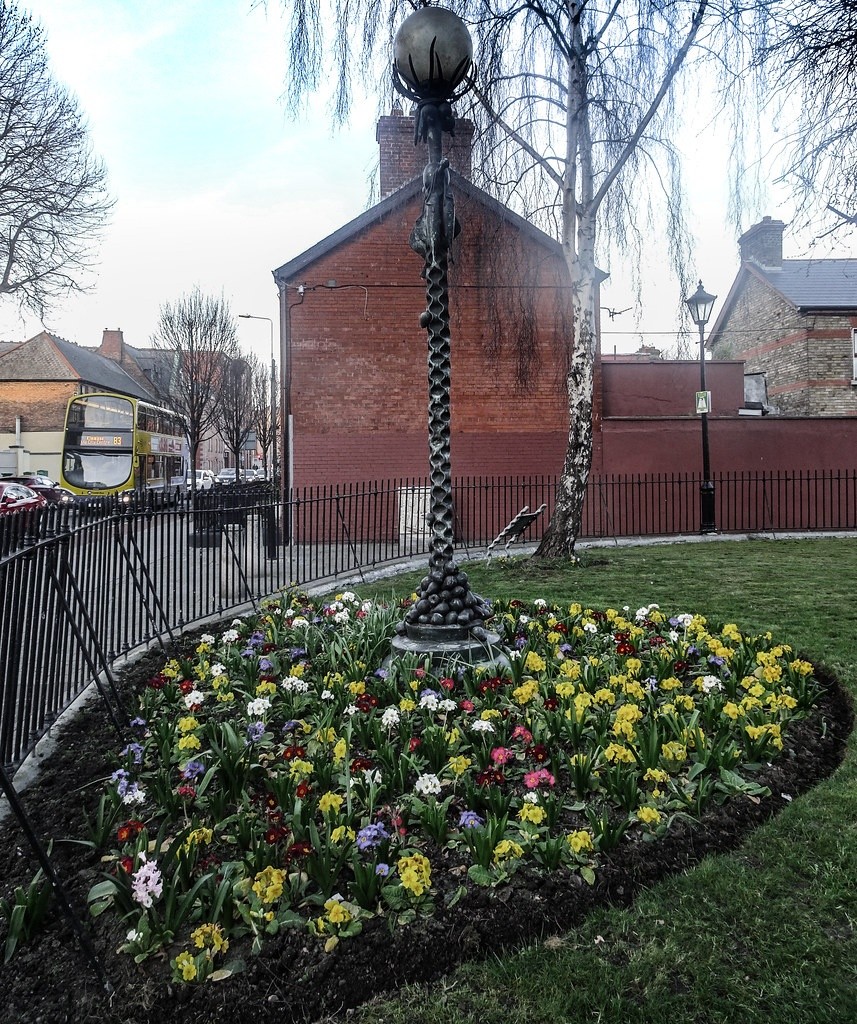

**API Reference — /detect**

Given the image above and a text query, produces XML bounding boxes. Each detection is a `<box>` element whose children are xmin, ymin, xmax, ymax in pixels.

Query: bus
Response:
<box><xmin>60</xmin><ymin>392</ymin><xmax>192</xmax><ymax>514</ymax></box>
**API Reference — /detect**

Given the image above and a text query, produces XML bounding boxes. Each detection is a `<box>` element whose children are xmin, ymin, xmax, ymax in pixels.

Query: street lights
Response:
<box><xmin>238</xmin><ymin>314</ymin><xmax>277</xmax><ymax>491</ymax></box>
<box><xmin>387</xmin><ymin>8</ymin><xmax>504</xmax><ymax>662</ymax></box>
<box><xmin>684</xmin><ymin>278</ymin><xmax>720</xmax><ymax>535</ymax></box>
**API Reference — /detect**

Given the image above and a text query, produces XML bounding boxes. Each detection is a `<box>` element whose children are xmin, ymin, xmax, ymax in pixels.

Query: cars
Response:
<box><xmin>186</xmin><ymin>467</ymin><xmax>274</xmax><ymax>499</ymax></box>
<box><xmin>0</xmin><ymin>475</ymin><xmax>76</xmax><ymax>535</ymax></box>
